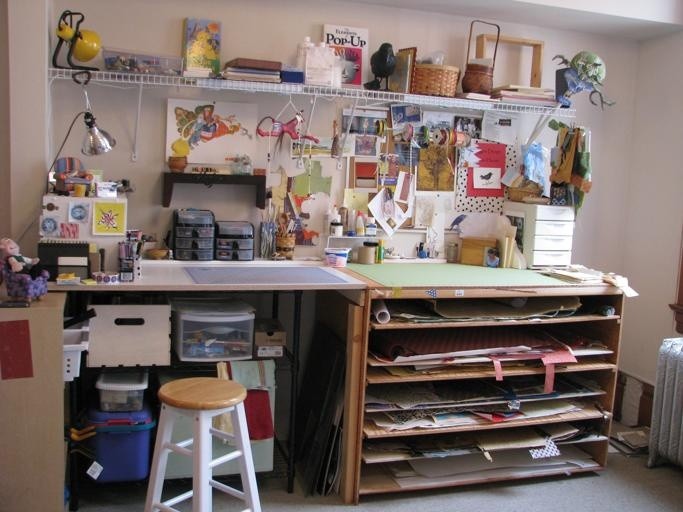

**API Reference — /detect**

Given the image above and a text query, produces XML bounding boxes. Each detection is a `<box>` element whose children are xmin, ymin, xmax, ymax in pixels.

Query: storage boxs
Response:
<box><xmin>85</xmin><ymin>296</ymin><xmax>192</xmax><ymax>373</ymax></box>
<box><xmin>176</xmin><ymin>301</ymin><xmax>255</xmax><ymax>360</ymax></box>
<box><xmin>83</xmin><ymin>410</ymin><xmax>154</xmax><ymax>482</ymax></box>
<box><xmin>95</xmin><ymin>372</ymin><xmax>147</xmax><ymax>411</ymax></box>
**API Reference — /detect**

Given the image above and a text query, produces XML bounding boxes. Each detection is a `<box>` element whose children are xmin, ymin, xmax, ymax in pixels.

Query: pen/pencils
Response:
<box><xmin>118</xmin><ymin>238</ymin><xmax>142</xmax><ymax>258</ymax></box>
<box><xmin>259</xmin><ymin>197</ymin><xmax>278</xmax><ymax>260</ymax></box>
<box><xmin>274</xmin><ymin>214</ymin><xmax>298</xmax><ymax>234</ymax></box>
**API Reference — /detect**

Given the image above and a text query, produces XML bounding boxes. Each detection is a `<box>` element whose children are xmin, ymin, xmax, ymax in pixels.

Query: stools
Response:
<box><xmin>143</xmin><ymin>378</ymin><xmax>262</xmax><ymax>512</ymax></box>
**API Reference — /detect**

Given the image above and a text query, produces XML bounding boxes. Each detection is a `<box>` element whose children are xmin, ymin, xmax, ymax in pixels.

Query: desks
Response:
<box><xmin>67</xmin><ymin>255</ymin><xmax>365</xmax><ymax>504</ymax></box>
<box><xmin>1</xmin><ymin>291</ymin><xmax>71</xmax><ymax>512</ymax></box>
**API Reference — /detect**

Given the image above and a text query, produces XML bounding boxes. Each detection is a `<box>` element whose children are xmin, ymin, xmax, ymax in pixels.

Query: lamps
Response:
<box><xmin>52</xmin><ymin>8</ymin><xmax>103</xmax><ymax>74</ymax></box>
<box><xmin>50</xmin><ymin>110</ymin><xmax>116</xmax><ymax>171</ymax></box>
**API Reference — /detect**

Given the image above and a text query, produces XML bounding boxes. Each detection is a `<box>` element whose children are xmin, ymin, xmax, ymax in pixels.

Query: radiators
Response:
<box><xmin>647</xmin><ymin>336</ymin><xmax>683</xmax><ymax>468</ymax></box>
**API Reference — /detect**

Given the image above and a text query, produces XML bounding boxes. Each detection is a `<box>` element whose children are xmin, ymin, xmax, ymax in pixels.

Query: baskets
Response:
<box><xmin>507</xmin><ymin>175</ymin><xmax>543</xmax><ymax>201</ymax></box>
<box><xmin>461</xmin><ymin>20</ymin><xmax>500</xmax><ymax>94</ymax></box>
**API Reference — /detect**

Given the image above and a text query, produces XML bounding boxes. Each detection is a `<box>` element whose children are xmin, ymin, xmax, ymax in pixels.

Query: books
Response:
<box><xmin>224</xmin><ymin>56</ymin><xmax>281</xmax><ymax>84</ymax></box>
<box><xmin>183</xmin><ymin>16</ymin><xmax>222</xmax><ymax>78</ymax></box>
<box><xmin>540</xmin><ymin>267</ymin><xmax>602</xmax><ymax>286</ymax></box>
<box><xmin>323</xmin><ymin>23</ymin><xmax>369</xmax><ymax>89</ymax></box>
<box><xmin>491</xmin><ymin>85</ymin><xmax>557</xmax><ymax>107</ymax></box>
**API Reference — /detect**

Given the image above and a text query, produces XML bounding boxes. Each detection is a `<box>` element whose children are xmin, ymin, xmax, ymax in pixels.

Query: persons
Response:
<box><xmin>486</xmin><ymin>249</ymin><xmax>499</xmax><ymax>267</ymax></box>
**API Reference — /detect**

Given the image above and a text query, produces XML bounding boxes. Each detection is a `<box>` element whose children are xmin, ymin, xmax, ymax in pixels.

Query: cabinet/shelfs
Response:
<box><xmin>342</xmin><ymin>260</ymin><xmax>627</xmax><ymax>503</ymax></box>
<box><xmin>43</xmin><ymin>1</ymin><xmax>579</xmax><ymax>171</ymax></box>
<box><xmin>504</xmin><ymin>201</ymin><xmax>576</xmax><ymax>270</ymax></box>
<box><xmin>163</xmin><ymin>171</ymin><xmax>265</xmax><ymax>208</ymax></box>
